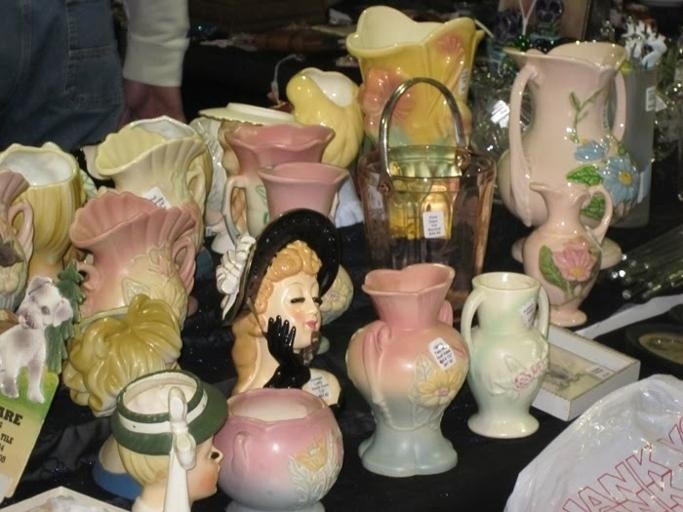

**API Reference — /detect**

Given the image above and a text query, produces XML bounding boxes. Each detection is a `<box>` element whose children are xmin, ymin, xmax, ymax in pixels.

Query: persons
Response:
<box><xmin>0</xmin><ymin>0</ymin><xmax>190</xmax><ymax>156</ymax></box>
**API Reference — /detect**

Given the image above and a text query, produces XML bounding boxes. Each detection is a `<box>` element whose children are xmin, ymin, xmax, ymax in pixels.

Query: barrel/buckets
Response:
<box><xmin>356</xmin><ymin>74</ymin><xmax>497</xmax><ymax>325</ymax></box>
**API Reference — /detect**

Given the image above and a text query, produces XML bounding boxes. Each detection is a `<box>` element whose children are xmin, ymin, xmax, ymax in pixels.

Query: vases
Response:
<box><xmin>1</xmin><ymin>6</ymin><xmax>659</xmax><ymax>511</ymax></box>
